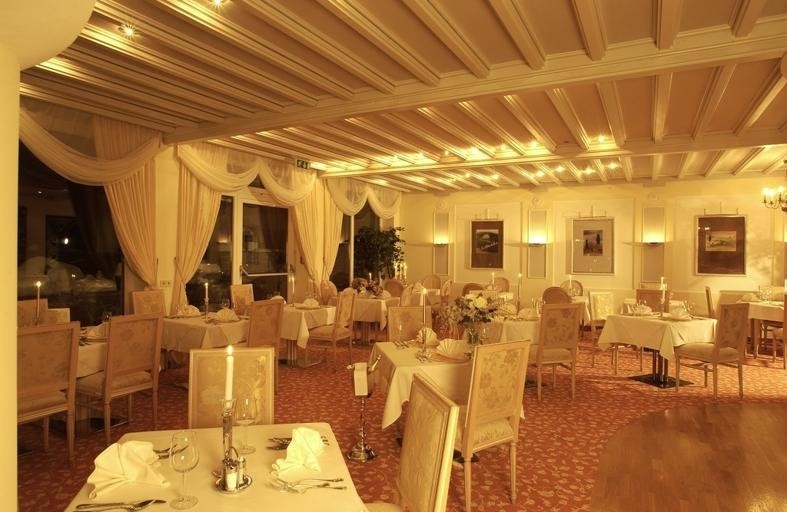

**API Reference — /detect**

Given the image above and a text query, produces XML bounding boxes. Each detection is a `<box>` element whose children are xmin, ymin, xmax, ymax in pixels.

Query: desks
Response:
<box><xmin>602</xmin><ymin>311</ymin><xmax>718</xmax><ymax>389</ymax></box>
<box><xmin>737</xmin><ymin>299</ymin><xmax>786</xmax><ymax>362</ymax></box>
<box><xmin>487</xmin><ymin>313</ymin><xmax>546</xmax><ymax>388</ymax></box>
<box><xmin>281</xmin><ymin>300</ymin><xmax>336</xmax><ymax>367</ymax></box>
<box><xmin>344</xmin><ymin>294</ymin><xmax>400</xmax><ymax>347</ymax></box>
<box><xmin>62</xmin><ymin>420</ymin><xmax>372</xmax><ymax>512</ymax></box>
<box><xmin>369</xmin><ymin>338</ymin><xmax>526</xmax><ymax>463</ymax></box>
<box><xmin>156</xmin><ymin>311</ymin><xmax>250</xmax><ymax>393</ymax></box>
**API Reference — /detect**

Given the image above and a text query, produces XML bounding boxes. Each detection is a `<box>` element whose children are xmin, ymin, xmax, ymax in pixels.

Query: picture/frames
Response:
<box><xmin>469</xmin><ymin>219</ymin><xmax>505</xmax><ymax>271</ymax></box>
<box><xmin>695</xmin><ymin>214</ymin><xmax>748</xmax><ymax>277</ymax></box>
<box><xmin>570</xmin><ymin>217</ymin><xmax>617</xmax><ymax>276</ymax></box>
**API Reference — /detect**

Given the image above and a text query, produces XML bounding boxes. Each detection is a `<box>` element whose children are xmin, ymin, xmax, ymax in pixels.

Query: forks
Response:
<box><xmin>270</xmin><ymin>475</ymin><xmax>346</xmax><ymax>495</ymax></box>
<box><xmin>153</xmin><ymin>443</ymin><xmax>179</xmax><ymax>454</ymax></box>
<box><xmin>159</xmin><ymin>443</ymin><xmax>190</xmax><ymax>460</ymax></box>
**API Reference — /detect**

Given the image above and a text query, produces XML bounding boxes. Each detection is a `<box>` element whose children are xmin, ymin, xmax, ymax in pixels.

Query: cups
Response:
<box><xmin>221</xmin><ymin>298</ymin><xmax>230</xmax><ymax>308</ymax></box>
<box><xmin>530</xmin><ymin>297</ymin><xmax>545</xmax><ymax>318</ymax></box>
<box><xmin>102</xmin><ymin>311</ymin><xmax>112</xmax><ymax>323</ymax></box>
<box><xmin>629</xmin><ymin>297</ymin><xmax>697</xmax><ymax>320</ymax></box>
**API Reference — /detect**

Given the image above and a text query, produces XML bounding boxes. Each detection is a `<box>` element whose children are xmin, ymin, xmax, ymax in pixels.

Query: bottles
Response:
<box><xmin>223</xmin><ymin>453</ymin><xmax>246</xmax><ymax>490</ymax></box>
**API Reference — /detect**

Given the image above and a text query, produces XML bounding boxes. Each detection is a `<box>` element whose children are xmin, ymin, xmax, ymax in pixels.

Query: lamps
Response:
<box><xmin>761</xmin><ymin>160</ymin><xmax>787</xmax><ymax>212</ymax></box>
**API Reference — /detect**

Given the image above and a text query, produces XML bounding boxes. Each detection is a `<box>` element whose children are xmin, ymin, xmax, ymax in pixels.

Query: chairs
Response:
<box><xmin>17</xmin><ymin>321</ymin><xmax>82</xmax><ymax>469</ymax></box>
<box><xmin>187</xmin><ymin>344</ymin><xmax>278</xmax><ymax>427</ymax></box>
<box><xmin>78</xmin><ymin>313</ymin><xmax>163</xmax><ymax>444</ymax></box>
<box><xmin>454</xmin><ymin>339</ymin><xmax>532</xmax><ymax>511</ymax></box>
<box><xmin>129</xmin><ymin>290</ymin><xmax>168</xmax><ymax>317</ymax></box>
<box><xmin>387</xmin><ymin>305</ymin><xmax>433</xmax><ymax>341</ymax></box>
<box><xmin>363</xmin><ymin>372</ymin><xmax>462</xmax><ymax>512</ymax></box>
<box><xmin>249</xmin><ymin>298</ymin><xmax>285</xmax><ymax>396</ymax></box>
<box><xmin>226</xmin><ymin>276</ymin><xmax>786</xmax><ymax>401</ymax></box>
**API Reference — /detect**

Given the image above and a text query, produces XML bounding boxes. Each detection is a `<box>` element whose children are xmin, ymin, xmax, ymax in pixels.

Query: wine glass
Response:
<box><xmin>234</xmin><ymin>393</ymin><xmax>258</xmax><ymax>454</ymax></box>
<box><xmin>461</xmin><ymin>317</ymin><xmax>490</xmax><ymax>345</ymax></box>
<box><xmin>170</xmin><ymin>429</ymin><xmax>199</xmax><ymax>509</ymax></box>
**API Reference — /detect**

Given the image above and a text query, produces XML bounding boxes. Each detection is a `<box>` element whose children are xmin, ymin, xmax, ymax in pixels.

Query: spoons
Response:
<box><xmin>75</xmin><ymin>497</ymin><xmax>164</xmax><ymax>511</ymax></box>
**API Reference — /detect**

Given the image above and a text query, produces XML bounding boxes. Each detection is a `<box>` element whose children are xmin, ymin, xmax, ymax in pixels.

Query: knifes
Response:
<box><xmin>264</xmin><ymin>435</ymin><xmax>330</xmax><ymax>448</ymax></box>
<box><xmin>75</xmin><ymin>498</ymin><xmax>167</xmax><ymax>508</ymax></box>
